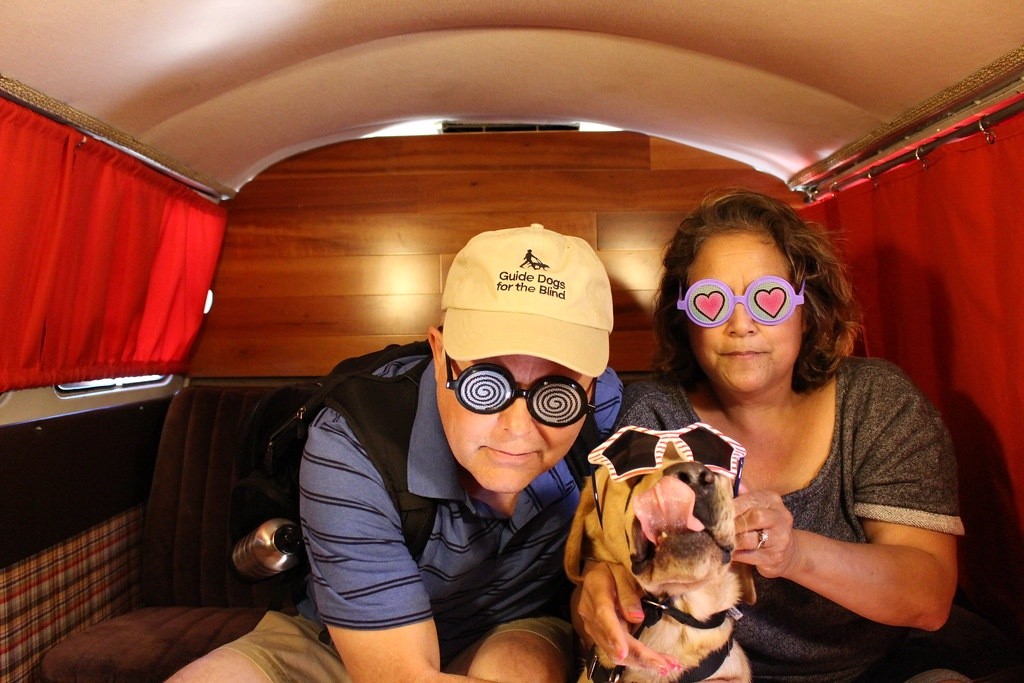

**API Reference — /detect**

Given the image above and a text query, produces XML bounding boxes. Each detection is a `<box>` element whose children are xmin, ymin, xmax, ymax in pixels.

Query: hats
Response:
<box><xmin>441</xmin><ymin>223</ymin><xmax>614</xmax><ymax>378</ymax></box>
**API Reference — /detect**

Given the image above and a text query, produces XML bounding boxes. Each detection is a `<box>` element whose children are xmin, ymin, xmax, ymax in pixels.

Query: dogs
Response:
<box><xmin>564</xmin><ymin>439</ymin><xmax>757</xmax><ymax>683</ymax></box>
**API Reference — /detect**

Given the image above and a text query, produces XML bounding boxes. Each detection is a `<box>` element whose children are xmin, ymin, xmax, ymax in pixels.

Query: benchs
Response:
<box><xmin>39</xmin><ymin>368</ymin><xmax>667</xmax><ymax>683</ymax></box>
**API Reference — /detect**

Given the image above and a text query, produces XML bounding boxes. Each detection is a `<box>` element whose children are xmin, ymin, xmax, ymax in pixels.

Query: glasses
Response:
<box><xmin>588</xmin><ymin>422</ymin><xmax>746</xmax><ymax>530</ymax></box>
<box><xmin>444</xmin><ymin>350</ymin><xmax>598</xmax><ymax>428</ymax></box>
<box><xmin>674</xmin><ymin>275</ymin><xmax>806</xmax><ymax>327</ymax></box>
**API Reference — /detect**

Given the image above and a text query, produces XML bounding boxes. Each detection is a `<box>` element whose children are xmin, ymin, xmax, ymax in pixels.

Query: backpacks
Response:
<box><xmin>227</xmin><ymin>339</ymin><xmax>597</xmax><ymax>586</ymax></box>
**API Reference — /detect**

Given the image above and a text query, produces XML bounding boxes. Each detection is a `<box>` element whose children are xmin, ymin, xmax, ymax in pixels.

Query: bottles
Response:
<box><xmin>233</xmin><ymin>519</ymin><xmax>305</xmax><ymax>580</ymax></box>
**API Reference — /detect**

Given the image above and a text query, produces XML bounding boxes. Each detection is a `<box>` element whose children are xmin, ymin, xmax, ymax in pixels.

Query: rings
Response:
<box><xmin>755</xmin><ymin>527</ymin><xmax>769</xmax><ymax>550</ymax></box>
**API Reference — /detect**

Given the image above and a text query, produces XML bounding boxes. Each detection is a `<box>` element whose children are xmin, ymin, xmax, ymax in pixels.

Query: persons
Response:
<box><xmin>578</xmin><ymin>192</ymin><xmax>977</xmax><ymax>683</ymax></box>
<box><xmin>164</xmin><ymin>227</ymin><xmax>624</xmax><ymax>683</ymax></box>
